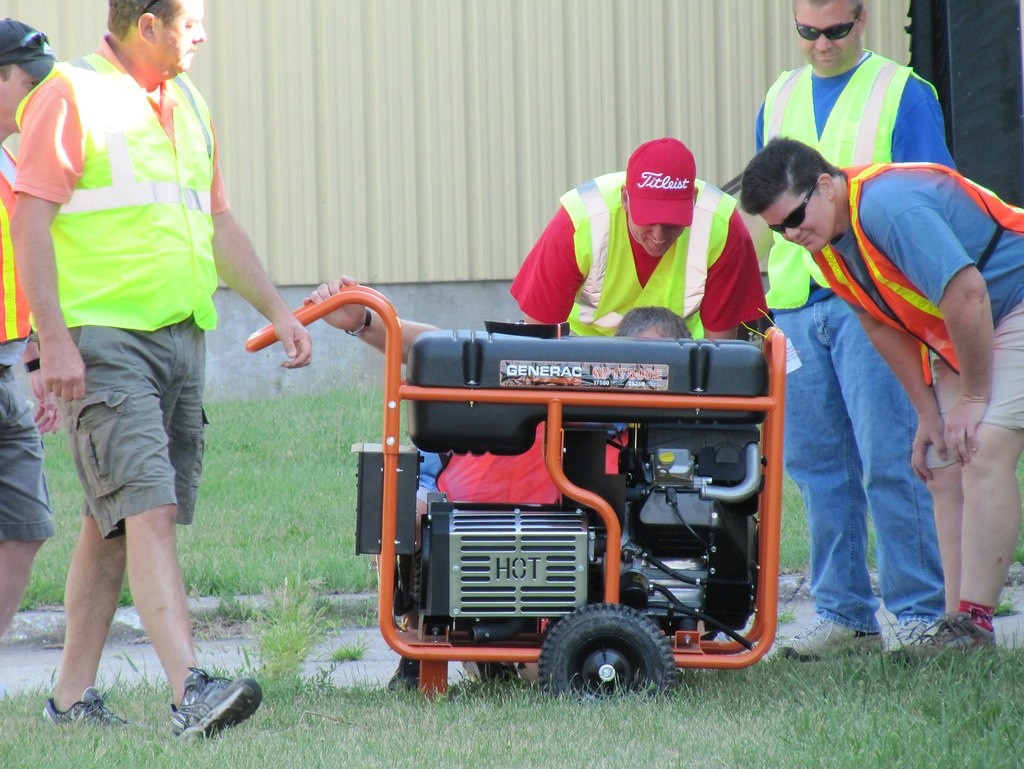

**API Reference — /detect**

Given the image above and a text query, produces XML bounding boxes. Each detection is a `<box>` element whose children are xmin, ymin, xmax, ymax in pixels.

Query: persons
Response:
<box><xmin>754</xmin><ymin>0</ymin><xmax>960</xmax><ymax>657</ymax></box>
<box><xmin>507</xmin><ymin>138</ymin><xmax>771</xmax><ymax>343</ymax></box>
<box><xmin>10</xmin><ymin>0</ymin><xmax>310</xmax><ymax>736</ymax></box>
<box><xmin>738</xmin><ymin>138</ymin><xmax>1024</xmax><ymax>662</ymax></box>
<box><xmin>0</xmin><ymin>17</ymin><xmax>58</xmax><ymax>638</ymax></box>
<box><xmin>304</xmin><ymin>276</ymin><xmax>694</xmax><ymax>689</ymax></box>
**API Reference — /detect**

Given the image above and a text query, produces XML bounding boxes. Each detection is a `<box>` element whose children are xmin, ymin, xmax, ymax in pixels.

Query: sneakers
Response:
<box><xmin>885</xmin><ymin>618</ymin><xmax>942</xmax><ymax>653</ymax></box>
<box><xmin>463</xmin><ymin>659</ymin><xmax>518</xmax><ymax>682</ymax></box>
<box><xmin>778</xmin><ymin>616</ymin><xmax>885</xmax><ymax>661</ymax></box>
<box><xmin>169</xmin><ymin>666</ymin><xmax>262</xmax><ymax>742</ymax></box>
<box><xmin>388</xmin><ymin>655</ymin><xmax>421</xmax><ymax>691</ymax></box>
<box><xmin>43</xmin><ymin>687</ymin><xmax>129</xmax><ymax>725</ymax></box>
<box><xmin>918</xmin><ymin>612</ymin><xmax>998</xmax><ymax>656</ymax></box>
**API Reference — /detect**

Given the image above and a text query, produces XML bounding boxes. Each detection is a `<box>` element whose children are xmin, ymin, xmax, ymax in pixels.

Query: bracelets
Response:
<box><xmin>23</xmin><ymin>357</ymin><xmax>41</xmax><ymax>373</ymax></box>
<box><xmin>345</xmin><ymin>309</ymin><xmax>372</xmax><ymax>336</ymax></box>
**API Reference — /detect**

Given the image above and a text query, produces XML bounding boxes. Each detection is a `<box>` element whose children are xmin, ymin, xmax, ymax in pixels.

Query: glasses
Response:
<box><xmin>794</xmin><ymin>6</ymin><xmax>863</xmax><ymax>41</ymax></box>
<box><xmin>0</xmin><ymin>31</ymin><xmax>50</xmax><ymax>56</ymax></box>
<box><xmin>766</xmin><ymin>177</ymin><xmax>818</xmax><ymax>234</ymax></box>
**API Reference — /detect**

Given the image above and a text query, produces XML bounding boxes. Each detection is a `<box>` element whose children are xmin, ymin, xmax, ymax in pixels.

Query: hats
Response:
<box><xmin>0</xmin><ymin>18</ymin><xmax>58</xmax><ymax>81</ymax></box>
<box><xmin>626</xmin><ymin>137</ymin><xmax>695</xmax><ymax>227</ymax></box>
